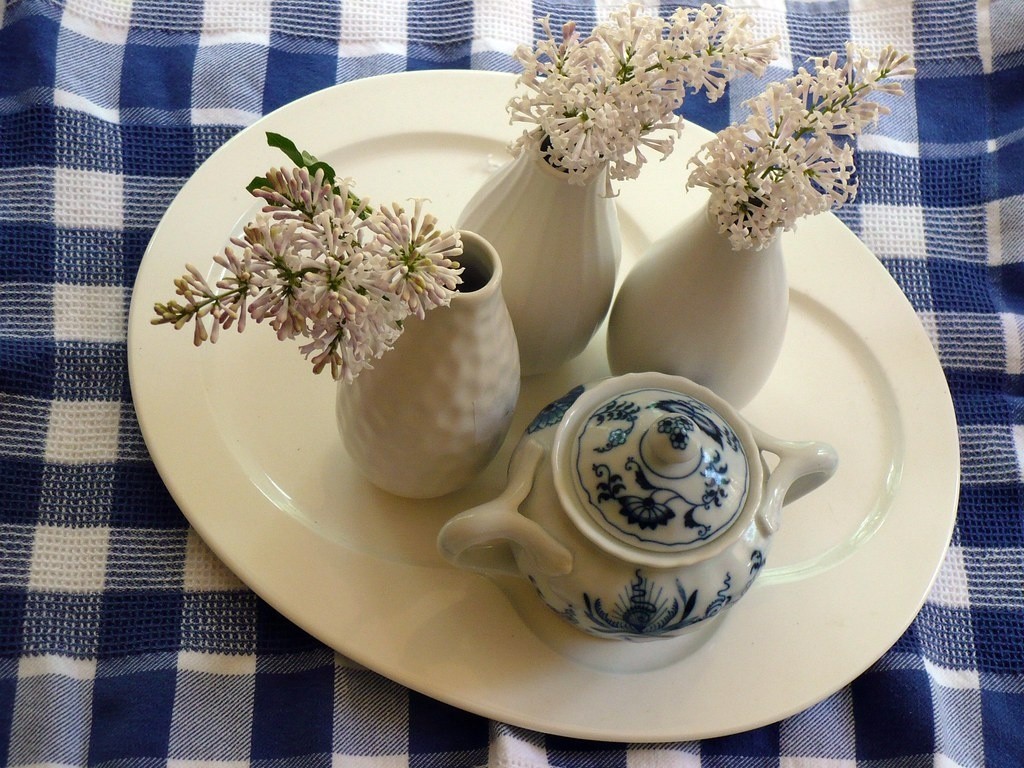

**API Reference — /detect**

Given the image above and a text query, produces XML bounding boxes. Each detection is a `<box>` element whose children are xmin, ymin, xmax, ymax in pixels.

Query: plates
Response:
<box><xmin>126</xmin><ymin>70</ymin><xmax>961</xmax><ymax>744</ymax></box>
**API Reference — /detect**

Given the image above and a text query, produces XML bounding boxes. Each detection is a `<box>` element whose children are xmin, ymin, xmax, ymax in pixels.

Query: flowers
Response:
<box><xmin>147</xmin><ymin>131</ymin><xmax>466</xmax><ymax>384</ymax></box>
<box><xmin>503</xmin><ymin>0</ymin><xmax>780</xmax><ymax>198</ymax></box>
<box><xmin>686</xmin><ymin>40</ymin><xmax>916</xmax><ymax>249</ymax></box>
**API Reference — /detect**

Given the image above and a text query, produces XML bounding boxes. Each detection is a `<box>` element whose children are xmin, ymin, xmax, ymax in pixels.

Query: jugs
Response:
<box><xmin>435</xmin><ymin>372</ymin><xmax>840</xmax><ymax>639</ymax></box>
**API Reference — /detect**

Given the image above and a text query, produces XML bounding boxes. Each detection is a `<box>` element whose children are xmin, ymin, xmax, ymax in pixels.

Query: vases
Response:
<box><xmin>456</xmin><ymin>141</ymin><xmax>622</xmax><ymax>376</ymax></box>
<box><xmin>336</xmin><ymin>231</ymin><xmax>520</xmax><ymax>498</ymax></box>
<box><xmin>607</xmin><ymin>196</ymin><xmax>791</xmax><ymax>412</ymax></box>
